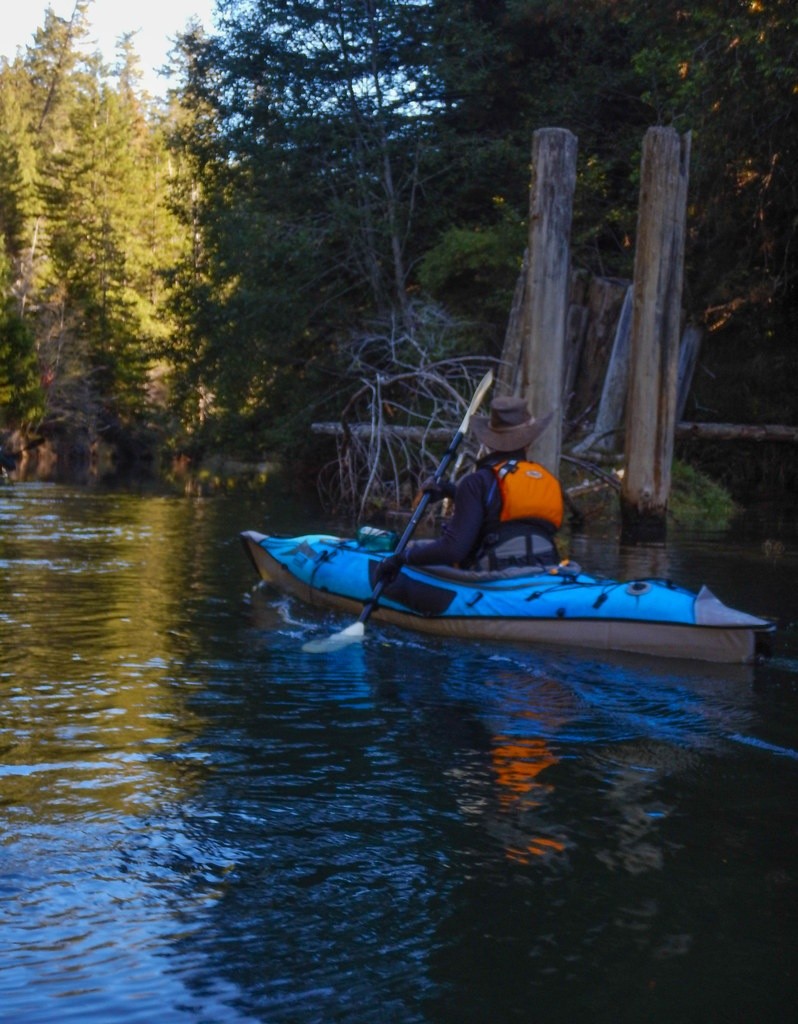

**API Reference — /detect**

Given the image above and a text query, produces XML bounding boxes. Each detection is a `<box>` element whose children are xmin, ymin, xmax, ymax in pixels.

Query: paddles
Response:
<box><xmin>301</xmin><ymin>370</ymin><xmax>496</xmax><ymax>652</ymax></box>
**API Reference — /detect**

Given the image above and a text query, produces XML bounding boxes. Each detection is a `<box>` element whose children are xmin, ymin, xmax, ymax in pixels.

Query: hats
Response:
<box><xmin>469</xmin><ymin>396</ymin><xmax>555</xmax><ymax>451</ymax></box>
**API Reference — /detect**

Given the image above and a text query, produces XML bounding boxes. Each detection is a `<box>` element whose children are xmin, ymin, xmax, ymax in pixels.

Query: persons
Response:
<box><xmin>374</xmin><ymin>397</ymin><xmax>562</xmax><ymax>583</ymax></box>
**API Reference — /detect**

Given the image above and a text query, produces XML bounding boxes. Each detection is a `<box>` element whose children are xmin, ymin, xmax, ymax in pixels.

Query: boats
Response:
<box><xmin>235</xmin><ymin>523</ymin><xmax>780</xmax><ymax>666</ymax></box>
